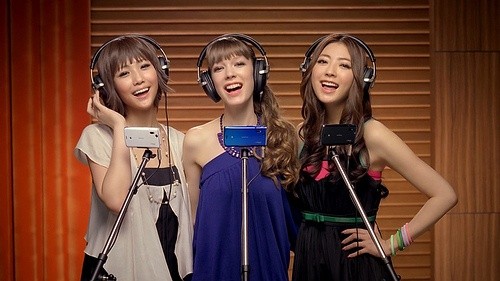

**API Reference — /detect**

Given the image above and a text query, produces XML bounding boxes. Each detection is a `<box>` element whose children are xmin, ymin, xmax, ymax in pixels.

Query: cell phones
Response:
<box><xmin>320</xmin><ymin>124</ymin><xmax>356</xmax><ymax>146</ymax></box>
<box><xmin>224</xmin><ymin>126</ymin><xmax>268</xmax><ymax>147</ymax></box>
<box><xmin>124</xmin><ymin>127</ymin><xmax>160</xmax><ymax>148</ymax></box>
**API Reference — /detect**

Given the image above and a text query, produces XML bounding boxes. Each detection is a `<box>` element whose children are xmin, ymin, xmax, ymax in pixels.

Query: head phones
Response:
<box><xmin>299</xmin><ymin>33</ymin><xmax>377</xmax><ymax>94</ymax></box>
<box><xmin>197</xmin><ymin>33</ymin><xmax>269</xmax><ymax>103</ymax></box>
<box><xmin>89</xmin><ymin>35</ymin><xmax>170</xmax><ymax>105</ymax></box>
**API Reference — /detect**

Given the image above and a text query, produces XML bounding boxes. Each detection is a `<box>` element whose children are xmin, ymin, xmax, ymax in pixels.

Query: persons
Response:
<box><xmin>183</xmin><ymin>38</ymin><xmax>298</xmax><ymax>281</ymax></box>
<box><xmin>72</xmin><ymin>35</ymin><xmax>194</xmax><ymax>281</ymax></box>
<box><xmin>289</xmin><ymin>33</ymin><xmax>458</xmax><ymax>281</ymax></box>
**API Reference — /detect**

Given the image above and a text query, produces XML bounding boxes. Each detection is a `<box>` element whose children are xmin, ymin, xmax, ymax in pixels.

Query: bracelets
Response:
<box><xmin>391</xmin><ymin>223</ymin><xmax>412</xmax><ymax>256</ymax></box>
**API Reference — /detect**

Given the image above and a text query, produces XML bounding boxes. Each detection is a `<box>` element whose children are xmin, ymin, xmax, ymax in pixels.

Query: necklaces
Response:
<box><xmin>217</xmin><ymin>113</ymin><xmax>262</xmax><ymax>159</ymax></box>
<box><xmin>131</xmin><ymin>130</ymin><xmax>179</xmax><ymax>204</ymax></box>
